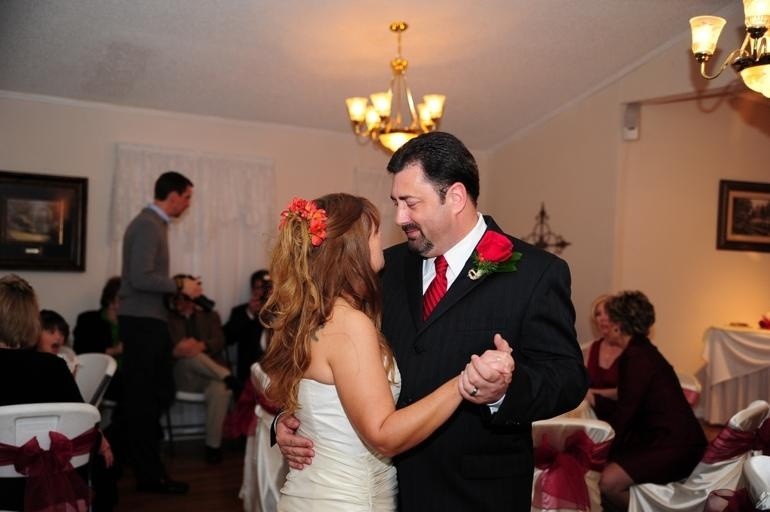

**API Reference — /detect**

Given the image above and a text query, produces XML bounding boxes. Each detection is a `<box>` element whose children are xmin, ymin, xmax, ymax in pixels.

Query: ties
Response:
<box><xmin>423</xmin><ymin>254</ymin><xmax>449</xmax><ymax>321</ymax></box>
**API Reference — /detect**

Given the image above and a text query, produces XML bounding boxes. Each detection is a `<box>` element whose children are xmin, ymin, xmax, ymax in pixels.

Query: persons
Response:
<box><xmin>587</xmin><ymin>295</ymin><xmax>623</xmax><ymax>426</ymax></box>
<box><xmin>270</xmin><ymin>130</ymin><xmax>588</xmax><ymax>511</ymax></box>
<box><xmin>0</xmin><ymin>273</ymin><xmax>85</xmax><ymax>512</ymax></box>
<box><xmin>119</xmin><ymin>171</ymin><xmax>195</xmax><ymax>495</ymax></box>
<box><xmin>598</xmin><ymin>289</ymin><xmax>708</xmax><ymax>511</ymax></box>
<box><xmin>267</xmin><ymin>193</ymin><xmax>511</xmax><ymax>511</ymax></box>
<box><xmin>73</xmin><ymin>277</ymin><xmax>127</xmax><ymax>355</ymax></box>
<box><xmin>221</xmin><ymin>269</ymin><xmax>271</xmax><ymax>392</ymax></box>
<box><xmin>37</xmin><ymin>310</ymin><xmax>71</xmax><ymax>355</ymax></box>
<box><xmin>170</xmin><ymin>272</ymin><xmax>235</xmax><ymax>463</ymax></box>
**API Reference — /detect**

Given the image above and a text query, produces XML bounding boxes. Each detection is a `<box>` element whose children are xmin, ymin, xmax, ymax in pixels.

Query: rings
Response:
<box><xmin>469</xmin><ymin>386</ymin><xmax>480</xmax><ymax>397</ymax></box>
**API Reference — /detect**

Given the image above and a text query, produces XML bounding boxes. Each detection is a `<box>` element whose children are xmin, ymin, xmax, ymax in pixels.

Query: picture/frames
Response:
<box><xmin>0</xmin><ymin>169</ymin><xmax>88</xmax><ymax>272</ymax></box>
<box><xmin>716</xmin><ymin>180</ymin><xmax>770</xmax><ymax>250</ymax></box>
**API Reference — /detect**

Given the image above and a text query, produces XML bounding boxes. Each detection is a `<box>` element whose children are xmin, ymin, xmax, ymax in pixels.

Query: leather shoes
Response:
<box><xmin>136</xmin><ymin>469</ymin><xmax>192</xmax><ymax>496</ymax></box>
<box><xmin>230</xmin><ymin>377</ymin><xmax>248</xmax><ymax>402</ymax></box>
<box><xmin>203</xmin><ymin>442</ymin><xmax>225</xmax><ymax>464</ymax></box>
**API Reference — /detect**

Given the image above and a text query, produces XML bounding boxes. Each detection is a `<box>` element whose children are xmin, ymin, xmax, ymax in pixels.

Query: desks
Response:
<box><xmin>702</xmin><ymin>325</ymin><xmax>770</xmax><ymax>426</ymax></box>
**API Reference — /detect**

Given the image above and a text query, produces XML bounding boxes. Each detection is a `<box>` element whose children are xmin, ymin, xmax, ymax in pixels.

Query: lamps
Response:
<box><xmin>345</xmin><ymin>21</ymin><xmax>447</xmax><ymax>154</ymax></box>
<box><xmin>689</xmin><ymin>0</ymin><xmax>770</xmax><ymax>102</ymax></box>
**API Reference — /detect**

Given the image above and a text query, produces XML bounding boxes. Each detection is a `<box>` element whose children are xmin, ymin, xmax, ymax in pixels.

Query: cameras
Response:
<box><xmin>258</xmin><ymin>273</ymin><xmax>273</xmax><ymax>306</ymax></box>
<box><xmin>173</xmin><ymin>274</ymin><xmax>215</xmax><ymax>313</ymax></box>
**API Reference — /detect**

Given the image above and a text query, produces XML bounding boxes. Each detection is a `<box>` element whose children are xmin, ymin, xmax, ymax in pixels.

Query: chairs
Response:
<box><xmin>58</xmin><ymin>345</ymin><xmax>76</xmax><ymax>371</ymax></box>
<box><xmin>529</xmin><ymin>418</ymin><xmax>615</xmax><ymax>512</ymax></box>
<box><xmin>73</xmin><ymin>353</ymin><xmax>117</xmax><ymax>409</ymax></box>
<box><xmin>164</xmin><ymin>338</ymin><xmax>234</xmax><ymax>457</ymax></box>
<box><xmin>0</xmin><ymin>403</ymin><xmax>100</xmax><ymax>512</ymax></box>
<box><xmin>626</xmin><ymin>401</ymin><xmax>770</xmax><ymax>511</ymax></box>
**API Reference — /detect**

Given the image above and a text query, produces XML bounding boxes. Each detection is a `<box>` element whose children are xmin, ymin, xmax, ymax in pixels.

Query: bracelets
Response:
<box><xmin>174</xmin><ymin>278</ymin><xmax>183</xmax><ymax>289</ymax></box>
